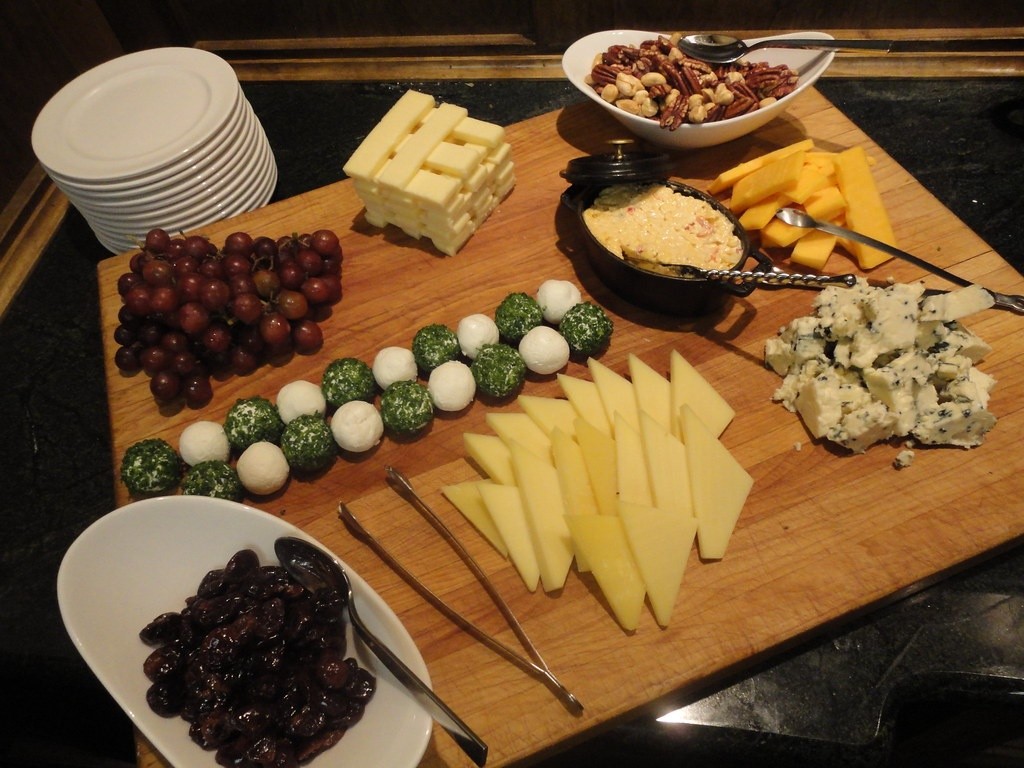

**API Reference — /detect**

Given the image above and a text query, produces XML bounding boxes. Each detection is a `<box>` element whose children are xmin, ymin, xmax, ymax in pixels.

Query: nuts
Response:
<box><xmin>585</xmin><ymin>32</ymin><xmax>799</xmax><ymax>131</ymax></box>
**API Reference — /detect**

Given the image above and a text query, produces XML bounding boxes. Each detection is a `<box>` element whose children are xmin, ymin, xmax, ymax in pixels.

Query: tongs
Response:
<box><xmin>339</xmin><ymin>466</ymin><xmax>586</xmax><ymax>716</ymax></box>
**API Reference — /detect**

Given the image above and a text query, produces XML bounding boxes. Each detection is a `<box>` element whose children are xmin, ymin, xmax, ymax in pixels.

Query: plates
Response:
<box><xmin>31</xmin><ymin>46</ymin><xmax>278</xmax><ymax>256</ymax></box>
<box><xmin>57</xmin><ymin>495</ymin><xmax>433</xmax><ymax>768</ymax></box>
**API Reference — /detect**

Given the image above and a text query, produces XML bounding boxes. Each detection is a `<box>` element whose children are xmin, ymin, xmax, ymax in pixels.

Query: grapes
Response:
<box><xmin>115</xmin><ymin>228</ymin><xmax>344</xmax><ymax>406</ymax></box>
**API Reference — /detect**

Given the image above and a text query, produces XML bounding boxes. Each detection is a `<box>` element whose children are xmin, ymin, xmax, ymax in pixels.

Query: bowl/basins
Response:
<box><xmin>559</xmin><ymin>177</ymin><xmax>772</xmax><ymax>318</ymax></box>
<box><xmin>561</xmin><ymin>24</ymin><xmax>836</xmax><ymax>149</ymax></box>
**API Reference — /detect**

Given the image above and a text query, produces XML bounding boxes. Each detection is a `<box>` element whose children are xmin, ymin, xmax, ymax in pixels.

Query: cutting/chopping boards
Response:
<box><xmin>97</xmin><ymin>88</ymin><xmax>1024</xmax><ymax>768</ymax></box>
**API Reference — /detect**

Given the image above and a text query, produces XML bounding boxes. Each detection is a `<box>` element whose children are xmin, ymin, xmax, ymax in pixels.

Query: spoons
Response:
<box><xmin>274</xmin><ymin>535</ymin><xmax>488</xmax><ymax>768</ymax></box>
<box><xmin>676</xmin><ymin>30</ymin><xmax>892</xmax><ymax>63</ymax></box>
